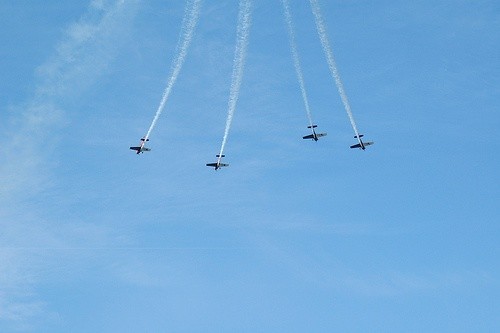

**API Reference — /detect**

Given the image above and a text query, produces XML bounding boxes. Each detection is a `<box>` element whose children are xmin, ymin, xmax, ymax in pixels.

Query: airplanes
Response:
<box><xmin>303</xmin><ymin>125</ymin><xmax>327</xmax><ymax>141</ymax></box>
<box><xmin>350</xmin><ymin>134</ymin><xmax>374</xmax><ymax>151</ymax></box>
<box><xmin>129</xmin><ymin>138</ymin><xmax>151</xmax><ymax>154</ymax></box>
<box><xmin>206</xmin><ymin>154</ymin><xmax>229</xmax><ymax>170</ymax></box>
